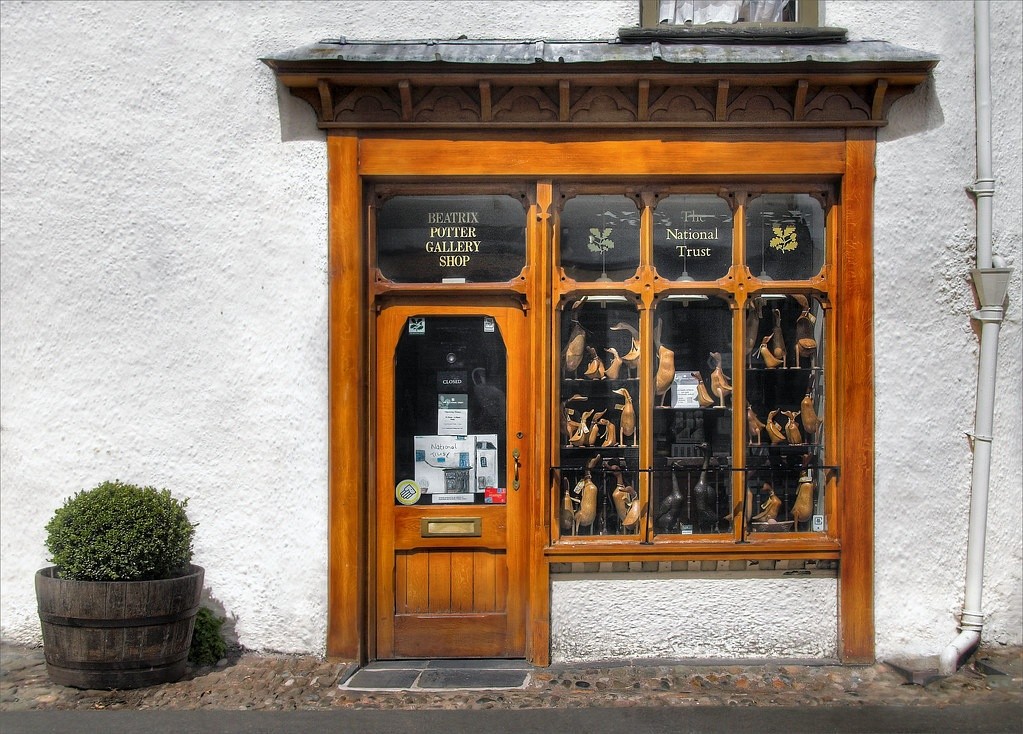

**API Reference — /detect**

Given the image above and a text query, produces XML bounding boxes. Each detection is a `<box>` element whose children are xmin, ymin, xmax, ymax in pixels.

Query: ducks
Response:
<box><xmin>561</xmin><ymin>294</ymin><xmax>824</xmax><ymax>535</ymax></box>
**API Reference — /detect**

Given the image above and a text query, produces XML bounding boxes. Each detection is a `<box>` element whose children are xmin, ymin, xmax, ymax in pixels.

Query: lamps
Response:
<box><xmin>594</xmin><ymin>195</ymin><xmax>613</xmax><ymax>282</ymax></box>
<box><xmin>756</xmin><ymin>193</ymin><xmax>772</xmax><ymax>281</ymax></box>
<box><xmin>674</xmin><ymin>196</ymin><xmax>695</xmax><ymax>281</ymax></box>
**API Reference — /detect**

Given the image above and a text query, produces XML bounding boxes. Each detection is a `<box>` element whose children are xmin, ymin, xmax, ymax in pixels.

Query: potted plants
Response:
<box><xmin>34</xmin><ymin>478</ymin><xmax>209</xmax><ymax>689</ymax></box>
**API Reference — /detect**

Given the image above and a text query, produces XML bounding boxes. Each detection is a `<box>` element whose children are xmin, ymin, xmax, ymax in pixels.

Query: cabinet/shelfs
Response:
<box><xmin>562</xmin><ymin>293</ymin><xmax>828</xmax><ymax>534</ymax></box>
